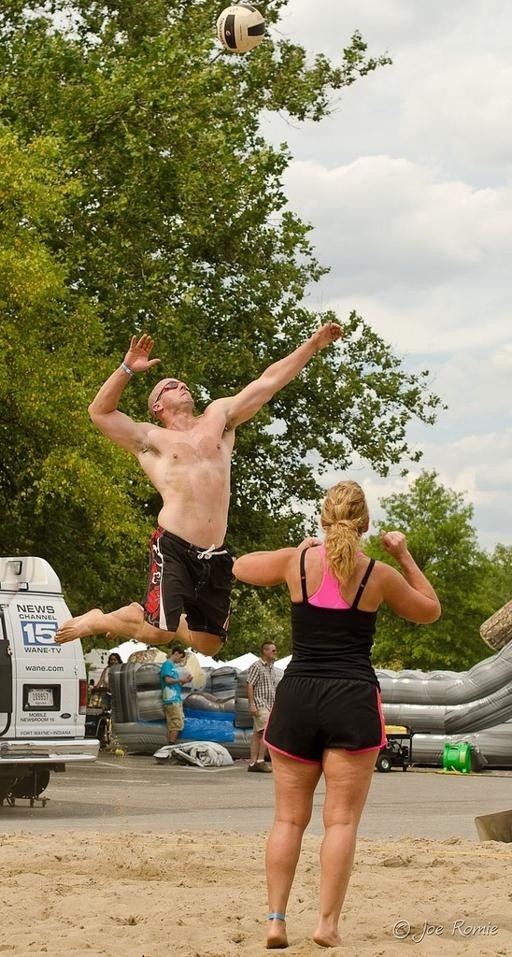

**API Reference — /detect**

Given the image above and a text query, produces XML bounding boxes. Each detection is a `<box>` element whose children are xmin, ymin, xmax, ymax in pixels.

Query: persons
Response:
<box><xmin>245</xmin><ymin>641</ymin><xmax>279</xmax><ymax>772</ymax></box>
<box><xmin>160</xmin><ymin>645</ymin><xmax>194</xmax><ymax>746</ymax></box>
<box><xmin>93</xmin><ymin>652</ymin><xmax>124</xmax><ymax>729</ymax></box>
<box><xmin>54</xmin><ymin>319</ymin><xmax>345</xmax><ymax>659</ymax></box>
<box><xmin>231</xmin><ymin>478</ymin><xmax>443</xmax><ymax>951</ymax></box>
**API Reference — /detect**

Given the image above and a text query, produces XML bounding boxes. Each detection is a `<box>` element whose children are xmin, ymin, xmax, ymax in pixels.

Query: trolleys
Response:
<box><xmin>376</xmin><ymin>725</ymin><xmax>416</xmax><ymax>772</ymax></box>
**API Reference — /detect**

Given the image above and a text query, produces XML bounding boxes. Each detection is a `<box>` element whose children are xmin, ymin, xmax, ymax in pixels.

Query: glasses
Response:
<box><xmin>155</xmin><ymin>382</ymin><xmax>191</xmax><ymax>413</ymax></box>
<box><xmin>272</xmin><ymin>650</ymin><xmax>279</xmax><ymax>652</ymax></box>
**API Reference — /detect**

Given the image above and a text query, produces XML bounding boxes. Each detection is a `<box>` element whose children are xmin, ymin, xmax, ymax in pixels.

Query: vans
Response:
<box><xmin>0</xmin><ymin>556</ymin><xmax>101</xmax><ymax>807</ymax></box>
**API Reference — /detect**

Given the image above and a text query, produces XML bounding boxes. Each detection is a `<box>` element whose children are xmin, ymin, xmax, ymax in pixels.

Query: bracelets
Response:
<box><xmin>120</xmin><ymin>363</ymin><xmax>139</xmax><ymax>379</ymax></box>
<box><xmin>249</xmin><ymin>702</ymin><xmax>256</xmax><ymax>706</ymax></box>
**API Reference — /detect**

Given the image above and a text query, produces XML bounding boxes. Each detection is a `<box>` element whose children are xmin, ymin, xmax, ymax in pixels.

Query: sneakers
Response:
<box><xmin>248</xmin><ymin>761</ymin><xmax>273</xmax><ymax>772</ymax></box>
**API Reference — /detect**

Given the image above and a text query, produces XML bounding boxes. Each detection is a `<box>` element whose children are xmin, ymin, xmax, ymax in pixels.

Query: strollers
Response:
<box><xmin>85</xmin><ymin>679</ymin><xmax>113</xmax><ymax>751</ymax></box>
